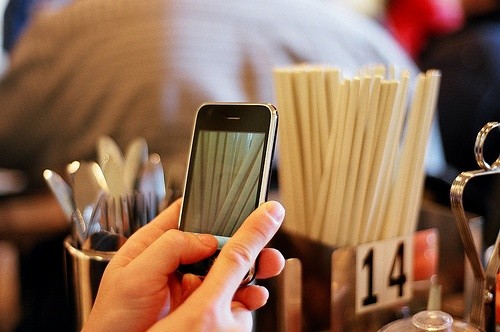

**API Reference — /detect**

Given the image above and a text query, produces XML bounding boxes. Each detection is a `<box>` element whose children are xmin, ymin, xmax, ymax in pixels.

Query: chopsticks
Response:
<box><xmin>271</xmin><ymin>64</ymin><xmax>442</xmax><ymax>249</ymax></box>
<box><xmin>181</xmin><ymin>134</ymin><xmax>265</xmax><ymax>237</ymax></box>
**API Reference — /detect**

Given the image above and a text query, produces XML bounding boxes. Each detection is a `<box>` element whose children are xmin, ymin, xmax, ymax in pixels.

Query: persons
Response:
<box><xmin>79</xmin><ymin>192</ymin><xmax>284</xmax><ymax>332</ymax></box>
<box><xmin>1</xmin><ymin>0</ymin><xmax>499</xmax><ymax>242</ymax></box>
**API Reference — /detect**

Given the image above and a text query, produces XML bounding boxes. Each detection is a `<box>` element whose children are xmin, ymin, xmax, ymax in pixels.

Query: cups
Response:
<box><xmin>62</xmin><ymin>237</ymin><xmax>124</xmax><ymax>332</ymax></box>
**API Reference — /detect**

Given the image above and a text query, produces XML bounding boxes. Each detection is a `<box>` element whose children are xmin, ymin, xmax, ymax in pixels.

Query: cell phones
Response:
<box><xmin>177</xmin><ymin>102</ymin><xmax>278</xmax><ymax>286</ymax></box>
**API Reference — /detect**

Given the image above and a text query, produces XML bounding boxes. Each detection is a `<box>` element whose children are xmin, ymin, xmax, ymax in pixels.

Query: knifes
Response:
<box><xmin>44</xmin><ymin>136</ymin><xmax>167</xmax><ymax>242</ymax></box>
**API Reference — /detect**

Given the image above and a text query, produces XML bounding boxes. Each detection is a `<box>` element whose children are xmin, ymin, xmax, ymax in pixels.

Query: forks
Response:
<box><xmin>74</xmin><ymin>188</ymin><xmax>159</xmax><ymax>251</ymax></box>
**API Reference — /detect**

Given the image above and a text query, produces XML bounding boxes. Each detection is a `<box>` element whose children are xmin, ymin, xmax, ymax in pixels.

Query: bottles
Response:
<box><xmin>377</xmin><ymin>309</ymin><xmax>486</xmax><ymax>332</ymax></box>
